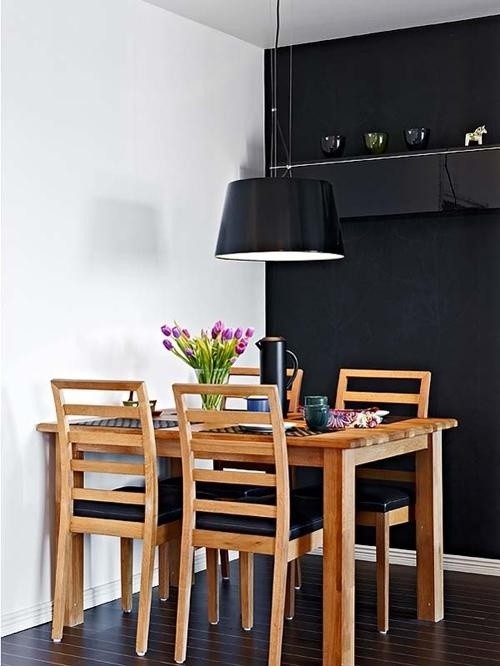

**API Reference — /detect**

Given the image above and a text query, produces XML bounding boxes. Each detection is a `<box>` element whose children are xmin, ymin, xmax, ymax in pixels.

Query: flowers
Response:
<box><xmin>159</xmin><ymin>318</ymin><xmax>255</xmax><ymax>409</ymax></box>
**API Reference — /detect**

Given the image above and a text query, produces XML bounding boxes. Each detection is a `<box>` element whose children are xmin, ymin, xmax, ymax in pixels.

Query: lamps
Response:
<box><xmin>214</xmin><ymin>0</ymin><xmax>348</xmax><ymax>266</ymax></box>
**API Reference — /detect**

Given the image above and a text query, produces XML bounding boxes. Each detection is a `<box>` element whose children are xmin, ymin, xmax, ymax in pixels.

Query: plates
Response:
<box><xmin>238</xmin><ymin>423</ymin><xmax>297</xmax><ymax>433</ymax></box>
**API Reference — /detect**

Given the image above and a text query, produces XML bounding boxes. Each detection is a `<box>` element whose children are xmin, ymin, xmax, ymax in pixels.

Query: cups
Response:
<box><xmin>363</xmin><ymin>132</ymin><xmax>388</xmax><ymax>155</ymax></box>
<box><xmin>402</xmin><ymin>128</ymin><xmax>431</xmax><ymax>151</ymax></box>
<box><xmin>304</xmin><ymin>395</ymin><xmax>329</xmax><ymax>430</ymax></box>
<box><xmin>245</xmin><ymin>397</ymin><xmax>270</xmax><ymax>412</ymax></box>
<box><xmin>321</xmin><ymin>135</ymin><xmax>346</xmax><ymax>158</ymax></box>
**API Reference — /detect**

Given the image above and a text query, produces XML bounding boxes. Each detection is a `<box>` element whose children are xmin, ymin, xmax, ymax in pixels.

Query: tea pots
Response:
<box><xmin>255</xmin><ymin>336</ymin><xmax>299</xmax><ymax>419</ymax></box>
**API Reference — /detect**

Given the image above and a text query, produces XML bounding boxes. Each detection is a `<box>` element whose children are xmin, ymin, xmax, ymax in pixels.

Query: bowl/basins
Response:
<box><xmin>121</xmin><ymin>400</ymin><xmax>156</xmax><ymax>412</ymax></box>
